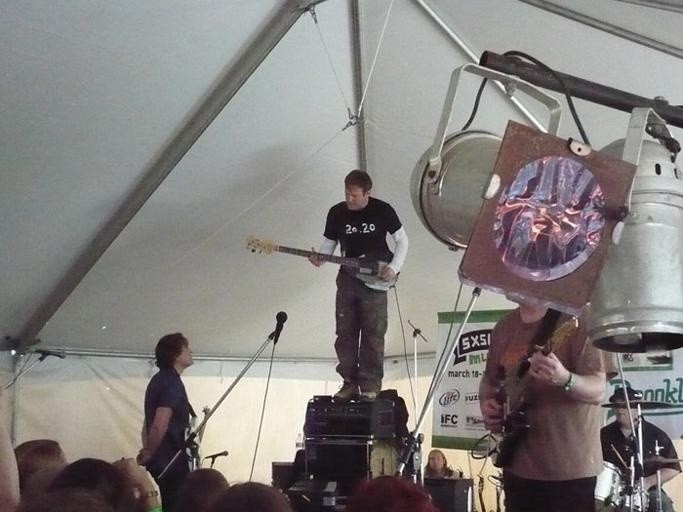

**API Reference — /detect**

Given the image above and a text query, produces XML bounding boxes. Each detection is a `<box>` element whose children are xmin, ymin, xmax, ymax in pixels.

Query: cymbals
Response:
<box><xmin>642</xmin><ymin>458</ymin><xmax>682</xmax><ymax>463</ymax></box>
<box><xmin>602</xmin><ymin>402</ymin><xmax>681</xmax><ymax>409</ymax></box>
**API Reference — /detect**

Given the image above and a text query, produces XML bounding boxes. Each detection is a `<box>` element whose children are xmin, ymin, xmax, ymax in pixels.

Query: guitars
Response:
<box><xmin>248</xmin><ymin>237</ymin><xmax>398</xmax><ymax>292</ymax></box>
<box><xmin>488</xmin><ymin>318</ymin><xmax>578</xmax><ymax>467</ymax></box>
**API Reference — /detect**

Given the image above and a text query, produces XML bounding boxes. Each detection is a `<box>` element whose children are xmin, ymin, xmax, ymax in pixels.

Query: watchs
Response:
<box><xmin>563</xmin><ymin>373</ymin><xmax>575</xmax><ymax>392</ymax></box>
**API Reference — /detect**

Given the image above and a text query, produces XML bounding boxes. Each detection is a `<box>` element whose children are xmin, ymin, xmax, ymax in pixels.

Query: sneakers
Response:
<box><xmin>360</xmin><ymin>392</ymin><xmax>376</xmax><ymax>401</ymax></box>
<box><xmin>334</xmin><ymin>382</ymin><xmax>359</xmax><ymax>400</ymax></box>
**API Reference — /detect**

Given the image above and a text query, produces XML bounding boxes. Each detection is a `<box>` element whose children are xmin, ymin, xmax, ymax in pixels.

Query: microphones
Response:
<box><xmin>273</xmin><ymin>311</ymin><xmax>288</xmax><ymax>344</ymax></box>
<box><xmin>407</xmin><ymin>319</ymin><xmax>430</xmax><ymax>343</ymax></box>
<box><xmin>205</xmin><ymin>451</ymin><xmax>229</xmax><ymax>459</ymax></box>
<box><xmin>34</xmin><ymin>349</ymin><xmax>67</xmax><ymax>359</ymax></box>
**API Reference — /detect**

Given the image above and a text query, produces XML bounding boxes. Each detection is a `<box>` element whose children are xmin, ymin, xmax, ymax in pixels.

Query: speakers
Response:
<box><xmin>287</xmin><ymin>478</ymin><xmax>360</xmax><ymax>512</ymax></box>
<box><xmin>303</xmin><ymin>399</ymin><xmax>401</xmax><ymax>439</ymax></box>
<box><xmin>304</xmin><ymin>438</ymin><xmax>400</xmax><ymax>481</ymax></box>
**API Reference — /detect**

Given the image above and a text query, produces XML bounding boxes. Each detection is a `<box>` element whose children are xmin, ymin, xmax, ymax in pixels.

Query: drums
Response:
<box><xmin>623</xmin><ymin>487</ymin><xmax>649</xmax><ymax>511</ymax></box>
<box><xmin>594</xmin><ymin>462</ymin><xmax>621</xmax><ymax>511</ymax></box>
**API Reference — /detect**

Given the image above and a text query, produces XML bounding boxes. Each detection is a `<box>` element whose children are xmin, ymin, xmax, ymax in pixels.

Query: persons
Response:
<box><xmin>308</xmin><ymin>170</ymin><xmax>408</xmax><ymax>401</ymax></box>
<box><xmin>478</xmin><ymin>293</ymin><xmax>620</xmax><ymax>511</ymax></box>
<box><xmin>422</xmin><ymin>451</ymin><xmax>460</xmax><ymax>479</ymax></box>
<box><xmin>598</xmin><ymin>387</ymin><xmax>682</xmax><ymax>511</ymax></box>
<box><xmin>130</xmin><ymin>333</ymin><xmax>195</xmax><ymax>487</ymax></box>
